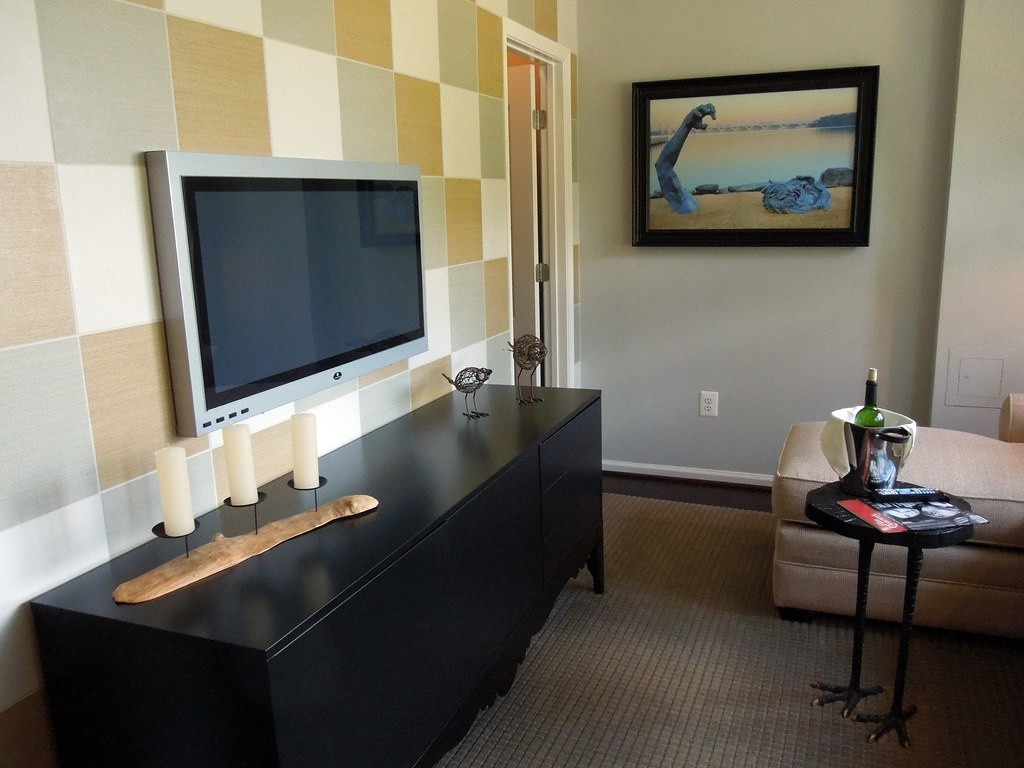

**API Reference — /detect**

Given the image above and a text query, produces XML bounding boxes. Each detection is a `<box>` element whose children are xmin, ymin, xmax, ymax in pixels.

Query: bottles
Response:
<box><xmin>855</xmin><ymin>368</ymin><xmax>884</xmax><ymax>427</ymax></box>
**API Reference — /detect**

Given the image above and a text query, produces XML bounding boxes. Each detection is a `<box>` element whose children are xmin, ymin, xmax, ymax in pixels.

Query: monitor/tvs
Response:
<box><xmin>146</xmin><ymin>150</ymin><xmax>429</xmax><ymax>437</ymax></box>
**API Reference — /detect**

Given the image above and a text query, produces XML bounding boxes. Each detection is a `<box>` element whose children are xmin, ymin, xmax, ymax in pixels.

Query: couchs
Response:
<box><xmin>772</xmin><ymin>394</ymin><xmax>1024</xmax><ymax>637</ymax></box>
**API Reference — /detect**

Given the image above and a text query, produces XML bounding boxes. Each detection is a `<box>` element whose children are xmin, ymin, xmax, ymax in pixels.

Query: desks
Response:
<box><xmin>803</xmin><ymin>476</ymin><xmax>973</xmax><ymax>747</ymax></box>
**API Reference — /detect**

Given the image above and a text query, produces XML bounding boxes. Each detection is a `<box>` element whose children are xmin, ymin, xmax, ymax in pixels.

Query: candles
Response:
<box><xmin>153</xmin><ymin>442</ymin><xmax>196</xmax><ymax>537</ymax></box>
<box><xmin>222</xmin><ymin>420</ymin><xmax>258</xmax><ymax>507</ymax></box>
<box><xmin>291</xmin><ymin>412</ymin><xmax>320</xmax><ymax>490</ymax></box>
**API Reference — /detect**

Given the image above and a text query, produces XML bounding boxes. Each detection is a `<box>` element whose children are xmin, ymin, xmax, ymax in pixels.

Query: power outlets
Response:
<box><xmin>699</xmin><ymin>390</ymin><xmax>718</xmax><ymax>417</ymax></box>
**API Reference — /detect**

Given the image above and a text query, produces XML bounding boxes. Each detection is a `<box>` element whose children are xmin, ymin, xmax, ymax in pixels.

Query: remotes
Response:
<box><xmin>874</xmin><ymin>487</ymin><xmax>944</xmax><ymax>504</ymax></box>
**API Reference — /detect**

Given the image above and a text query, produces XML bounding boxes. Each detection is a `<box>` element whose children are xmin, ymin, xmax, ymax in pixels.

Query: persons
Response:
<box><xmin>879</xmin><ymin>501</ymin><xmax>965</xmax><ymax>523</ymax></box>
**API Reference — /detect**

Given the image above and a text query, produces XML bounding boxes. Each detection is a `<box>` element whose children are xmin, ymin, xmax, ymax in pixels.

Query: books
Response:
<box><xmin>837</xmin><ymin>496</ymin><xmax>991</xmax><ymax>534</ymax></box>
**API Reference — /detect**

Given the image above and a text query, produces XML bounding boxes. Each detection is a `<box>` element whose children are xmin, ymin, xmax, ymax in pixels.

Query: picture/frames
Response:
<box><xmin>631</xmin><ymin>65</ymin><xmax>881</xmax><ymax>246</ymax></box>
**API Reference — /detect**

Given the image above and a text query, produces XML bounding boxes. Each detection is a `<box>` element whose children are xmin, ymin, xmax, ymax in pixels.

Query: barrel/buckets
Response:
<box><xmin>839</xmin><ymin>422</ymin><xmax>911</xmax><ymax>499</ymax></box>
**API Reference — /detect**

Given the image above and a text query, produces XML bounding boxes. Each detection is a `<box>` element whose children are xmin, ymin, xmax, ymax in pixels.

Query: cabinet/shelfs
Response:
<box><xmin>29</xmin><ymin>381</ymin><xmax>607</xmax><ymax>768</ymax></box>
